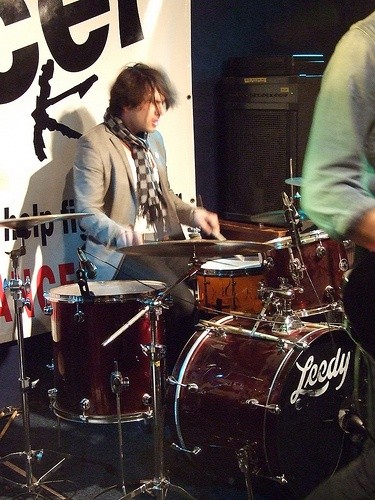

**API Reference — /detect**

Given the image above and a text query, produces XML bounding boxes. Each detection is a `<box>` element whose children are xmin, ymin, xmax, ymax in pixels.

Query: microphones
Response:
<box><xmin>77</xmin><ymin>248</ymin><xmax>97</xmax><ymax>279</ymax></box>
<box><xmin>283</xmin><ymin>191</ymin><xmax>304</xmax><ymax>228</ymax></box>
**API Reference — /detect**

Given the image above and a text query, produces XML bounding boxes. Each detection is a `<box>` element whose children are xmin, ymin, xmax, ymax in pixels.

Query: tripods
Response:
<box><xmin>0</xmin><ymin>230</ymin><xmax>71</xmax><ymax>500</ymax></box>
<box><xmin>102</xmin><ymin>249</ymin><xmax>200</xmax><ymax>500</ymax></box>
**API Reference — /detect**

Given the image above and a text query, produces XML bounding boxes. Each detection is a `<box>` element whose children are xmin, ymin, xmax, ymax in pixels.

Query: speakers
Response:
<box><xmin>218</xmin><ymin>74</ymin><xmax>322</xmax><ymax>225</ymax></box>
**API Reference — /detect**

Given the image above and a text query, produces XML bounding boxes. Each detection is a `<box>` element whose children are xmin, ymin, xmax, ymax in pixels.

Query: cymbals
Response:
<box><xmin>115</xmin><ymin>238</ymin><xmax>275</xmax><ymax>258</ymax></box>
<box><xmin>284</xmin><ymin>176</ymin><xmax>303</xmax><ymax>187</ymax></box>
<box><xmin>249</xmin><ymin>207</ymin><xmax>316</xmax><ymax>227</ymax></box>
<box><xmin>0</xmin><ymin>212</ymin><xmax>97</xmax><ymax>233</ymax></box>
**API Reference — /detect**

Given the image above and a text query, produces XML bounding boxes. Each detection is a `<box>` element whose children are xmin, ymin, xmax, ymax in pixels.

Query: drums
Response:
<box><xmin>195</xmin><ymin>257</ymin><xmax>270</xmax><ymax>319</ymax></box>
<box><xmin>170</xmin><ymin>316</ymin><xmax>357</xmax><ymax>493</ymax></box>
<box><xmin>45</xmin><ymin>279</ymin><xmax>171</xmax><ymax>423</ymax></box>
<box><xmin>259</xmin><ymin>230</ymin><xmax>351</xmax><ymax>318</ymax></box>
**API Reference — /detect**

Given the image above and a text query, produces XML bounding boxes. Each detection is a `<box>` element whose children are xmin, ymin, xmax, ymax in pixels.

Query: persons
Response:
<box><xmin>301</xmin><ymin>12</ymin><xmax>375</xmax><ymax>357</ymax></box>
<box><xmin>73</xmin><ymin>63</ymin><xmax>219</xmax><ymax>360</ymax></box>
